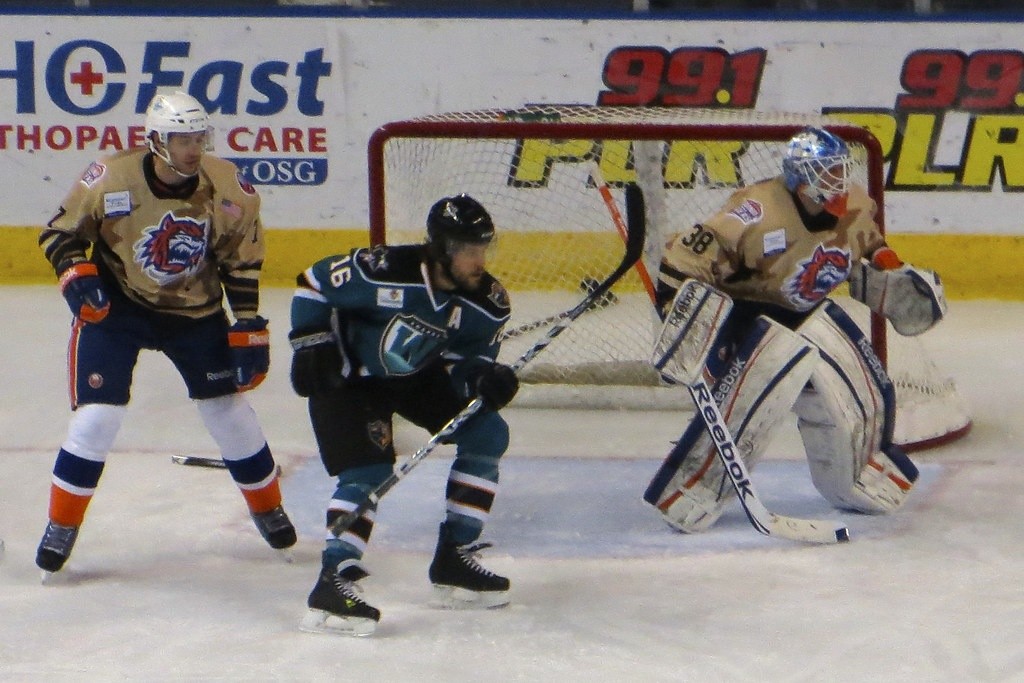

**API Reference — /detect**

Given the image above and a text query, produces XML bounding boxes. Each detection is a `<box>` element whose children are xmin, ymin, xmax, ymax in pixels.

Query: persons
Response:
<box><xmin>37</xmin><ymin>92</ymin><xmax>301</xmax><ymax>584</ymax></box>
<box><xmin>641</xmin><ymin>129</ymin><xmax>946</xmax><ymax>535</ymax></box>
<box><xmin>292</xmin><ymin>193</ymin><xmax>523</xmax><ymax>635</ymax></box>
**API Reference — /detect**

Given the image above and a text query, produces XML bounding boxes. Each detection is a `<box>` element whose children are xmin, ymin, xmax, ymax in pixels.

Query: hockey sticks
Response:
<box><xmin>579</xmin><ymin>158</ymin><xmax>850</xmax><ymax>544</ymax></box>
<box><xmin>172</xmin><ymin>455</ymin><xmax>292</xmax><ymax>477</ymax></box>
<box><xmin>501</xmin><ymin>276</ymin><xmax>622</xmax><ymax>341</ymax></box>
<box><xmin>326</xmin><ymin>182</ymin><xmax>647</xmax><ymax>540</ymax></box>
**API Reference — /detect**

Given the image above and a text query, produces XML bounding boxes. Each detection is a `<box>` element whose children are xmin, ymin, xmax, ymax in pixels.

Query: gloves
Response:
<box><xmin>55</xmin><ymin>258</ymin><xmax>110</xmax><ymax>323</ymax></box>
<box><xmin>228</xmin><ymin>315</ymin><xmax>270</xmax><ymax>392</ymax></box>
<box><xmin>289</xmin><ymin>321</ymin><xmax>344</xmax><ymax>399</ymax></box>
<box><xmin>478</xmin><ymin>363</ymin><xmax>519</xmax><ymax>411</ymax></box>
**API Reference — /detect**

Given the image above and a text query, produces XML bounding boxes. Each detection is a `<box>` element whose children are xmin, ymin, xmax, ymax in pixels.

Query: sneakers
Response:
<box><xmin>428</xmin><ymin>521</ymin><xmax>510</xmax><ymax>608</ymax></box>
<box><xmin>251</xmin><ymin>503</ymin><xmax>297</xmax><ymax>563</ymax></box>
<box><xmin>35</xmin><ymin>520</ymin><xmax>78</xmax><ymax>584</ymax></box>
<box><xmin>298</xmin><ymin>547</ymin><xmax>380</xmax><ymax>637</ymax></box>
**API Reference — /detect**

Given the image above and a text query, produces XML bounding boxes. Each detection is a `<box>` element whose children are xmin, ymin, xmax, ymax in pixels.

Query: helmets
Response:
<box><xmin>781</xmin><ymin>125</ymin><xmax>850</xmax><ymax>217</ymax></box>
<box><xmin>428</xmin><ymin>194</ymin><xmax>494</xmax><ymax>244</ymax></box>
<box><xmin>145</xmin><ymin>91</ymin><xmax>208</xmax><ymax>144</ymax></box>
